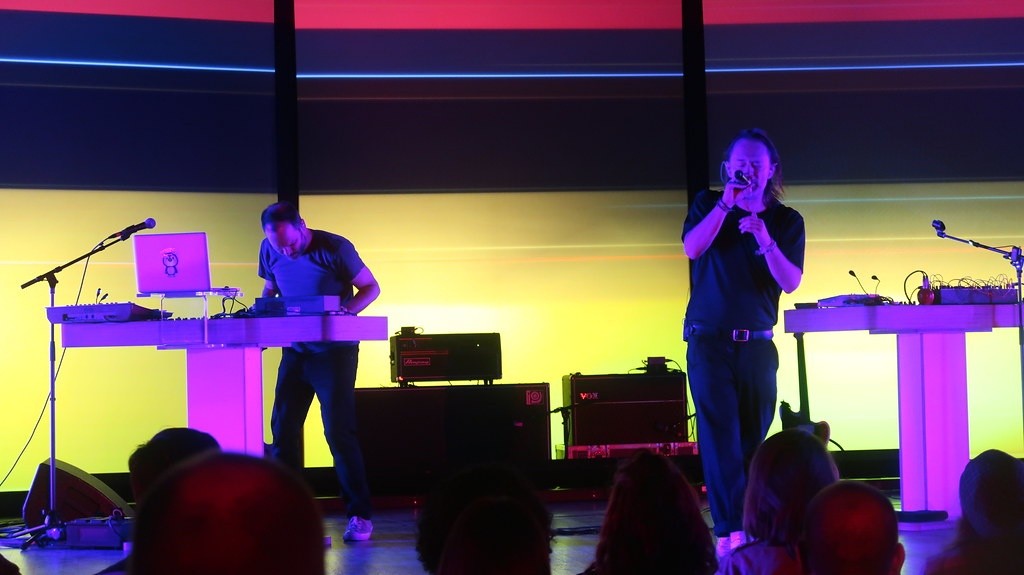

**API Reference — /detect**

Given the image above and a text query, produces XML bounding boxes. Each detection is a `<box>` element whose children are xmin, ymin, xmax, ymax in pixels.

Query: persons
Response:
<box><xmin>416</xmin><ymin>466</ymin><xmax>551</xmax><ymax>575</ymax></box>
<box><xmin>90</xmin><ymin>427</ymin><xmax>327</xmax><ymax>575</ymax></box>
<box><xmin>577</xmin><ymin>454</ymin><xmax>719</xmax><ymax>575</ymax></box>
<box><xmin>253</xmin><ymin>201</ymin><xmax>381</xmax><ymax>540</ymax></box>
<box><xmin>713</xmin><ymin>429</ymin><xmax>904</xmax><ymax>575</ymax></box>
<box><xmin>925</xmin><ymin>449</ymin><xmax>1024</xmax><ymax>575</ymax></box>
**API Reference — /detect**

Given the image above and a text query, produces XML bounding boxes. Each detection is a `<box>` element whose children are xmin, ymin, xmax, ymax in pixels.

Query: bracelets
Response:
<box><xmin>718</xmin><ymin>200</ymin><xmax>731</xmax><ymax>212</ymax></box>
<box><xmin>760</xmin><ymin>241</ymin><xmax>776</xmax><ymax>253</ymax></box>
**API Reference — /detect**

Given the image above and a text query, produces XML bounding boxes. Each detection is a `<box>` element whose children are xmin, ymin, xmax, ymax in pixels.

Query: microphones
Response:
<box><xmin>733</xmin><ymin>171</ymin><xmax>751</xmax><ymax>186</ymax></box>
<box><xmin>109</xmin><ymin>218</ymin><xmax>156</xmax><ymax>237</ymax></box>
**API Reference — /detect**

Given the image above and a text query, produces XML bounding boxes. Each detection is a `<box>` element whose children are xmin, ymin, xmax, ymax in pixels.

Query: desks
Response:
<box><xmin>62</xmin><ymin>316</ymin><xmax>388</xmax><ymax>456</ymax></box>
<box><xmin>783</xmin><ymin>302</ymin><xmax>1024</xmax><ymax>527</ymax></box>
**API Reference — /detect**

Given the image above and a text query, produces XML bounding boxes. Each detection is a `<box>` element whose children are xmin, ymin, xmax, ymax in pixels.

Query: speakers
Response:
<box><xmin>23</xmin><ymin>457</ymin><xmax>136</xmax><ymax>538</ymax></box>
<box><xmin>354</xmin><ymin>383</ymin><xmax>553</xmax><ymax>473</ymax></box>
<box><xmin>391</xmin><ymin>333</ymin><xmax>503</xmax><ymax>384</ymax></box>
<box><xmin>561</xmin><ymin>371</ymin><xmax>688</xmax><ymax>445</ymax></box>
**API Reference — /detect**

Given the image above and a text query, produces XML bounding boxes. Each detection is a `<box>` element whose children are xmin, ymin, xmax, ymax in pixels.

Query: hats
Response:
<box><xmin>592</xmin><ymin>454</ymin><xmax>721</xmax><ymax>575</ymax></box>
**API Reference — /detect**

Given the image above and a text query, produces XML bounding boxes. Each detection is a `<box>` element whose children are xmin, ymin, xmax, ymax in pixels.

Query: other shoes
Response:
<box><xmin>342</xmin><ymin>516</ymin><xmax>373</xmax><ymax>541</ymax></box>
<box><xmin>730</xmin><ymin>531</ymin><xmax>750</xmax><ymax>548</ymax></box>
<box><xmin>717</xmin><ymin>536</ymin><xmax>732</xmax><ymax>556</ymax></box>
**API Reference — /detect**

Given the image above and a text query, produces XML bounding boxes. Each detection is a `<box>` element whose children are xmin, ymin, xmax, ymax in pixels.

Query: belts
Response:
<box><xmin>688</xmin><ymin>324</ymin><xmax>774</xmax><ymax>342</ymax></box>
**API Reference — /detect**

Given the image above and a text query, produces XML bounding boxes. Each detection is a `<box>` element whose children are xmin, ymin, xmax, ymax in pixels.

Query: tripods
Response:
<box><xmin>6</xmin><ymin>235</ymin><xmax>133</xmax><ymax>549</ymax></box>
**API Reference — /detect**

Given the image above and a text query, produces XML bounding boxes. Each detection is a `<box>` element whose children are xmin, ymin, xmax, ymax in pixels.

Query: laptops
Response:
<box><xmin>133</xmin><ymin>232</ymin><xmax>240</xmax><ymax>291</ymax></box>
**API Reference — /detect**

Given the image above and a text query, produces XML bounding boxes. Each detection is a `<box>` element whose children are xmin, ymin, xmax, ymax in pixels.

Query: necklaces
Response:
<box><xmin>682</xmin><ymin>129</ymin><xmax>806</xmax><ymax>557</ymax></box>
